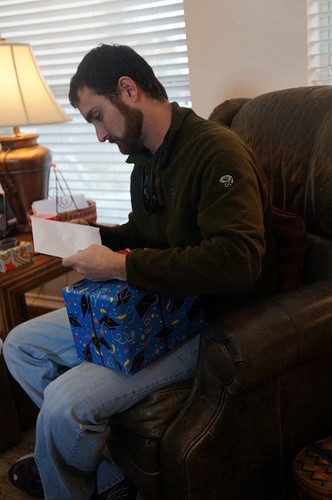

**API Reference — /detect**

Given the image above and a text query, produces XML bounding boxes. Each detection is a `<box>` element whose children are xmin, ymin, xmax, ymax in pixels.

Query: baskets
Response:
<box><xmin>25</xmin><ymin>162</ymin><xmax>97</xmax><ymax>229</ymax></box>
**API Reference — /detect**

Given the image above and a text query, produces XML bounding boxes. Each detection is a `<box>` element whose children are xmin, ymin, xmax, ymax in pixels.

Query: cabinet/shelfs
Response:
<box><xmin>0</xmin><ymin>222</ymin><xmax>119</xmax><ymax>454</ymax></box>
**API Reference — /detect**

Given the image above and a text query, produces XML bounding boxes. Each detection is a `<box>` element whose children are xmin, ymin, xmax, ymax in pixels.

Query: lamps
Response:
<box><xmin>0</xmin><ymin>43</ymin><xmax>73</xmax><ymax>233</ymax></box>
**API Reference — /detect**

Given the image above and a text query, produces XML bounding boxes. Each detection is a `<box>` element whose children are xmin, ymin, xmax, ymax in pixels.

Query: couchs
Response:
<box><xmin>108</xmin><ymin>85</ymin><xmax>332</xmax><ymax>500</ymax></box>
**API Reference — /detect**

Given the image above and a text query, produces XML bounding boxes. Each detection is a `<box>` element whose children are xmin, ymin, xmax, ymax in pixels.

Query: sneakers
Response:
<box><xmin>7</xmin><ymin>453</ymin><xmax>142</xmax><ymax>500</ymax></box>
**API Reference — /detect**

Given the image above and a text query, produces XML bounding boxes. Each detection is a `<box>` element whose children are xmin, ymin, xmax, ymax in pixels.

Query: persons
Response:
<box><xmin>3</xmin><ymin>44</ymin><xmax>271</xmax><ymax>500</ymax></box>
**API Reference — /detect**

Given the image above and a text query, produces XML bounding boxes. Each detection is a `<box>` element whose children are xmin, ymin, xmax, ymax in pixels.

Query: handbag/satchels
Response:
<box><xmin>253</xmin><ymin>205</ymin><xmax>306</xmax><ymax>289</ymax></box>
